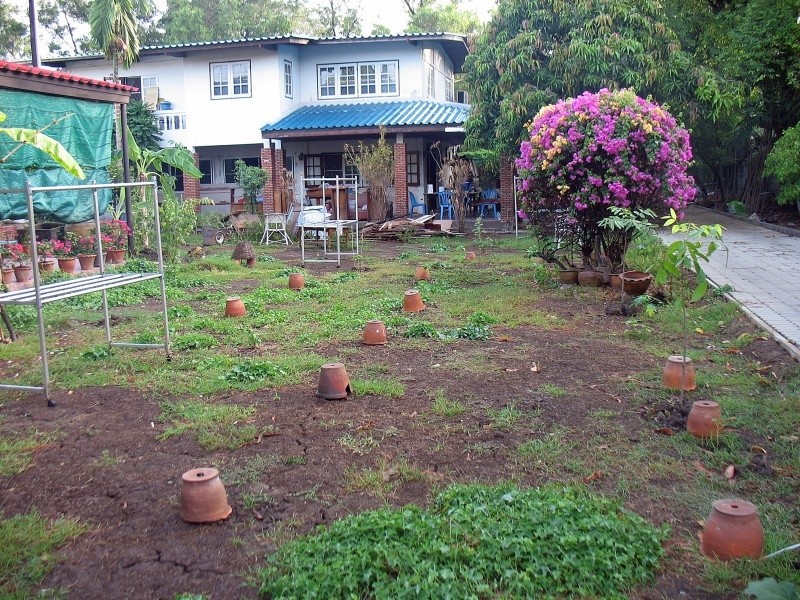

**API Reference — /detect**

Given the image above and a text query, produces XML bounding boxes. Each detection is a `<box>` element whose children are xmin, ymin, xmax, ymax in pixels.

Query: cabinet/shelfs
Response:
<box><xmin>0</xmin><ymin>175</ymin><xmax>171</xmax><ymax>406</ymax></box>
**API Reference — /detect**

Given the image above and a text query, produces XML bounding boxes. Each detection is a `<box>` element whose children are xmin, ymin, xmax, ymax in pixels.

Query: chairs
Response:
<box><xmin>410</xmin><ymin>192</ymin><xmax>426</xmax><ymax>217</ymax></box>
<box><xmin>439</xmin><ymin>184</ymin><xmax>498</xmax><ymax>219</ymax></box>
<box><xmin>259</xmin><ymin>213</ymin><xmax>294</xmax><ymax>245</ymax></box>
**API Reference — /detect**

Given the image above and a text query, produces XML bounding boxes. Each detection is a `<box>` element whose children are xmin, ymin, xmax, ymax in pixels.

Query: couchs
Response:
<box><xmin>310</xmin><ymin>193</ymin><xmax>368</xmax><ymax>220</ymax></box>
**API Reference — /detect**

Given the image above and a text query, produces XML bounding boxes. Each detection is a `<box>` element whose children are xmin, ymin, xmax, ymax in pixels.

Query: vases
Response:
<box><xmin>180</xmin><ymin>255</ymin><xmax>764</xmax><ymax>565</ymax></box>
<box><xmin>2</xmin><ymin>269</ymin><xmax>15</xmax><ymax>284</ymax></box>
<box><xmin>38</xmin><ymin>261</ymin><xmax>54</xmax><ymax>275</ymax></box>
<box><xmin>105</xmin><ymin>246</ymin><xmax>117</xmax><ymax>262</ymax></box>
<box><xmin>94</xmin><ymin>253</ymin><xmax>106</xmax><ymax>267</ymax></box>
<box><xmin>57</xmin><ymin>257</ymin><xmax>77</xmax><ymax>273</ymax></box>
<box><xmin>77</xmin><ymin>255</ymin><xmax>97</xmax><ymax>269</ymax></box>
<box><xmin>110</xmin><ymin>249</ymin><xmax>126</xmax><ymax>263</ymax></box>
<box><xmin>14</xmin><ymin>266</ymin><xmax>31</xmax><ymax>282</ymax></box>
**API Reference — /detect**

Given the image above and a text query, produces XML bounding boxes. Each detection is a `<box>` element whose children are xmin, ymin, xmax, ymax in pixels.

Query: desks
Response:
<box><xmin>231</xmin><ymin>202</ymin><xmax>262</xmax><ymax>214</ymax></box>
<box><xmin>423</xmin><ymin>191</ymin><xmax>481</xmax><ymax>215</ymax></box>
<box><xmin>306</xmin><ymin>188</ymin><xmax>347</xmax><ymax>220</ymax></box>
<box><xmin>296</xmin><ymin>222</ymin><xmax>355</xmax><ymax>249</ymax></box>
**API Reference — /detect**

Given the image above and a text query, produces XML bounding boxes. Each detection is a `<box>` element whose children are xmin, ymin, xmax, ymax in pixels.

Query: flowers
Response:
<box><xmin>0</xmin><ymin>220</ymin><xmax>133</xmax><ymax>268</ymax></box>
